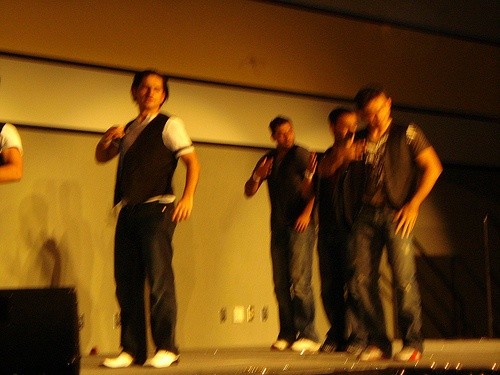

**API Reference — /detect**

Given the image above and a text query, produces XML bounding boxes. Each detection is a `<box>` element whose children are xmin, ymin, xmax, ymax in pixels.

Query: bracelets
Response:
<box><xmin>253</xmin><ymin>173</ymin><xmax>261</xmax><ymax>183</ymax></box>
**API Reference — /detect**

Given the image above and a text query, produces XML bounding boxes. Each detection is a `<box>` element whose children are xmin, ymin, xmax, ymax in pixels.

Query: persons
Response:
<box><xmin>354</xmin><ymin>84</ymin><xmax>444</xmax><ymax>362</ymax></box>
<box><xmin>313</xmin><ymin>106</ymin><xmax>369</xmax><ymax>354</ymax></box>
<box><xmin>245</xmin><ymin>116</ymin><xmax>318</xmax><ymax>351</ymax></box>
<box><xmin>95</xmin><ymin>69</ymin><xmax>201</xmax><ymax>368</ymax></box>
<box><xmin>0</xmin><ymin>122</ymin><xmax>25</xmax><ymax>183</ymax></box>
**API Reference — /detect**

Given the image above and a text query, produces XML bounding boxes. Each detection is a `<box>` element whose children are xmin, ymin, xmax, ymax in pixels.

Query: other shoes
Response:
<box><xmin>318</xmin><ymin>342</ymin><xmax>341</xmax><ymax>352</ymax></box>
<box><xmin>394</xmin><ymin>346</ymin><xmax>422</xmax><ymax>361</ymax></box>
<box><xmin>103</xmin><ymin>352</ymin><xmax>133</xmax><ymax>368</ymax></box>
<box><xmin>343</xmin><ymin>345</ymin><xmax>367</xmax><ymax>355</ymax></box>
<box><xmin>292</xmin><ymin>337</ymin><xmax>322</xmax><ymax>352</ymax></box>
<box><xmin>144</xmin><ymin>351</ymin><xmax>180</xmax><ymax>369</ymax></box>
<box><xmin>271</xmin><ymin>339</ymin><xmax>288</xmax><ymax>351</ymax></box>
<box><xmin>357</xmin><ymin>345</ymin><xmax>387</xmax><ymax>361</ymax></box>
